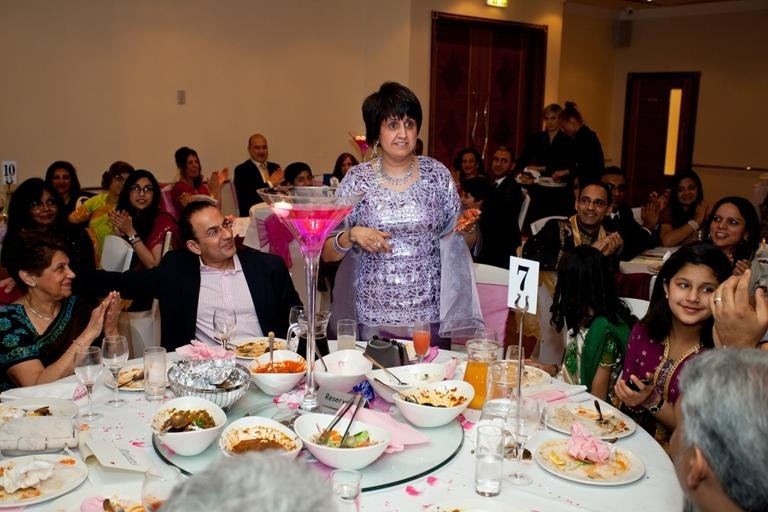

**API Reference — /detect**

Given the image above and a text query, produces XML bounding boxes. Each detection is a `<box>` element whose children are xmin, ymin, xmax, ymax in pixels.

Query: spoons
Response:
<box><xmin>594</xmin><ymin>400</ymin><xmax>609</xmax><ymax>424</ymax></box>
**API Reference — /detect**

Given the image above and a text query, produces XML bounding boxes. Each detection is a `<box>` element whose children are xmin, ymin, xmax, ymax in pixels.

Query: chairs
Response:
<box><xmin>449</xmin><ymin>185</ymin><xmax>662</xmax><ymax>354</ymax></box>
<box><xmin>72</xmin><ymin>178</ymin><xmax>309</xmax><ymax>367</ymax></box>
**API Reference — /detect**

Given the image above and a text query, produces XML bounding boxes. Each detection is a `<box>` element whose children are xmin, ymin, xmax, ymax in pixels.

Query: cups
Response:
<box><xmin>330</xmin><ymin>467</ymin><xmax>361</xmax><ymax>511</ymax></box>
<box><xmin>696</xmin><ymin>229</ymin><xmax>704</xmax><ymax>241</ymax></box>
<box><xmin>142</xmin><ymin>466</ymin><xmax>181</xmax><ymax>510</ymax></box>
<box><xmin>412</xmin><ymin>319</ymin><xmax>430</xmax><ymax>361</ymax></box>
<box><xmin>505</xmin><ymin>345</ymin><xmax>524</xmax><ymax>364</ymax></box>
<box><xmin>473</xmin><ymin>386</ymin><xmax>536</xmax><ymax>498</ymax></box>
<box><xmin>143</xmin><ymin>346</ymin><xmax>167</xmax><ymax>403</ymax></box>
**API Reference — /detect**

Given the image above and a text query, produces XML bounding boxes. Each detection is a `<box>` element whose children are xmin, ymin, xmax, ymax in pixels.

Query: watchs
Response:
<box><xmin>647</xmin><ymin>397</ymin><xmax>666</xmax><ymax>416</ymax></box>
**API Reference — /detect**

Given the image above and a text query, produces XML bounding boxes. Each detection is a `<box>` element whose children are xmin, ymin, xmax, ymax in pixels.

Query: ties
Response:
<box><xmin>261</xmin><ymin>163</ymin><xmax>270</xmax><ymax>183</ymax></box>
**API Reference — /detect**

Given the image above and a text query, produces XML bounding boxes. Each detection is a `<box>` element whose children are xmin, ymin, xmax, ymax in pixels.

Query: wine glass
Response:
<box><xmin>251</xmin><ymin>184</ymin><xmax>360</xmax><ymax>465</ymax></box>
<box><xmin>72</xmin><ymin>347</ymin><xmax>104</xmax><ymax>423</ymax></box>
<box><xmin>0</xmin><ymin>198</ymin><xmax>4</xmax><ymax>219</ymax></box>
<box><xmin>350</xmin><ymin>131</ymin><xmax>375</xmax><ymax>163</ymax></box>
<box><xmin>102</xmin><ymin>337</ymin><xmax>129</xmax><ymax>407</ymax></box>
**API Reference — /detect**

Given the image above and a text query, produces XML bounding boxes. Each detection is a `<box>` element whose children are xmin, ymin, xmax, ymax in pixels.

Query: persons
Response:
<box><xmin>548</xmin><ymin>244</ymin><xmax>642</xmax><ymax>408</ymax></box>
<box><xmin>319</xmin><ymin>77</ymin><xmax>488</xmax><ymax>352</ymax></box>
<box><xmin>154</xmin><ymin>447</ymin><xmax>338</xmax><ymax>512</ymax></box>
<box><xmin>1</xmin><ymin>146</ymin><xmax>307</xmax><ymax>388</ymax></box>
<box><xmin>612</xmin><ymin>240</ymin><xmax>734</xmax><ymax>458</ymax></box>
<box><xmin>667</xmin><ymin>345</ymin><xmax>766</xmax><ymax>512</ymax></box>
<box><xmin>523</xmin><ymin>164</ymin><xmax>768</xmax><ymax>348</ymax></box>
<box><xmin>233</xmin><ymin>134</ymin><xmax>362</xmax><ymax>218</ymax></box>
<box><xmin>415</xmin><ymin>99</ymin><xmax>604</xmax><ymax>269</ymax></box>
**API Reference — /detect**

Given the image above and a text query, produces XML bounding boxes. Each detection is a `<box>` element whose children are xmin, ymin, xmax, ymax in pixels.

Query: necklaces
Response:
<box><xmin>378</xmin><ymin>154</ymin><xmax>417</xmax><ymax>184</ymax></box>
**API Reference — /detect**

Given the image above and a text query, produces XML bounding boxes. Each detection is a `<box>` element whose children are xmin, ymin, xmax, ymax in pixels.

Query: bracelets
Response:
<box><xmin>553</xmin><ymin>363</ymin><xmax>561</xmax><ymax>376</ymax></box>
<box><xmin>333</xmin><ymin>229</ymin><xmax>352</xmax><ymax>254</ymax></box>
<box><xmin>468</xmin><ymin>223</ymin><xmax>477</xmax><ymax>233</ymax></box>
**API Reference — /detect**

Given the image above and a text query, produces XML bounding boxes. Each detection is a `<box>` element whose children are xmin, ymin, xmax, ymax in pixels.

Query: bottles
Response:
<box><xmin>463</xmin><ymin>340</ymin><xmax>505</xmax><ymax>410</ymax></box>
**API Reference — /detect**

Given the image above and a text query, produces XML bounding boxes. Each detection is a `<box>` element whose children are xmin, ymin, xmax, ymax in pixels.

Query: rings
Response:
<box><xmin>374</xmin><ymin>240</ymin><xmax>382</xmax><ymax>248</ymax></box>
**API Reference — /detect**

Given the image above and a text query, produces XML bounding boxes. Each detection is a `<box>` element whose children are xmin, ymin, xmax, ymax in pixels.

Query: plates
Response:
<box><xmin>362</xmin><ymin>363</ymin><xmax>448</xmax><ymax>405</ymax></box>
<box><xmin>393</xmin><ymin>379</ymin><xmax>474</xmax><ymax>428</ymax></box>
<box><xmin>540</xmin><ymin>405</ymin><xmax>637</xmax><ymax>440</ymax></box>
<box><xmin>3</xmin><ymin>453</ymin><xmax>88</xmax><ymax>508</ymax></box>
<box><xmin>107</xmin><ymin>364</ymin><xmax>171</xmax><ymax>388</ymax></box>
<box><xmin>514</xmin><ymin>173</ymin><xmax>539</xmax><ymax>185</ymax></box>
<box><xmin>224</xmin><ymin>336</ymin><xmax>251</xmax><ymax>357</ymax></box>
<box><xmin>648</xmin><ymin>260</ymin><xmax>665</xmax><ymax>273</ymax></box>
<box><xmin>535</xmin><ymin>439</ymin><xmax>644</xmax><ymax>488</ymax></box>
<box><xmin>1</xmin><ymin>398</ymin><xmax>80</xmax><ymax>423</ymax></box>
<box><xmin>167</xmin><ymin>359</ymin><xmax>251</xmax><ymax>407</ymax></box>
<box><xmin>150</xmin><ymin>396</ymin><xmax>228</xmax><ymax>457</ymax></box>
<box><xmin>392</xmin><ymin>338</ymin><xmax>432</xmax><ymax>359</ymax></box>
<box><xmin>487</xmin><ymin>363</ymin><xmax>552</xmax><ymax>388</ymax></box>
<box><xmin>218</xmin><ymin>416</ymin><xmax>302</xmax><ymax>466</ymax></box>
<box><xmin>294</xmin><ymin>413</ymin><xmax>390</xmax><ymax>468</ymax></box>
<box><xmin>536</xmin><ymin>177</ymin><xmax>568</xmax><ymax>187</ymax></box>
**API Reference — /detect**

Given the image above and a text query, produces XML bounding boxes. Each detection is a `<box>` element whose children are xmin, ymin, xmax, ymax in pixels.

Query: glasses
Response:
<box><xmin>28</xmin><ymin>198</ymin><xmax>57</xmax><ymax>209</ymax></box>
<box><xmin>197</xmin><ymin>218</ymin><xmax>234</xmax><ymax>241</ymax></box>
<box><xmin>130</xmin><ymin>184</ymin><xmax>153</xmax><ymax>194</ymax></box>
<box><xmin>577</xmin><ymin>197</ymin><xmax>609</xmax><ymax>210</ymax></box>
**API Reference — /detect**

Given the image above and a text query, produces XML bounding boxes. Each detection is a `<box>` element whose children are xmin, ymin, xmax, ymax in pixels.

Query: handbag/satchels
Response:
<box><xmin>366</xmin><ymin>335</ymin><xmax>411</xmax><ymax>370</ymax></box>
